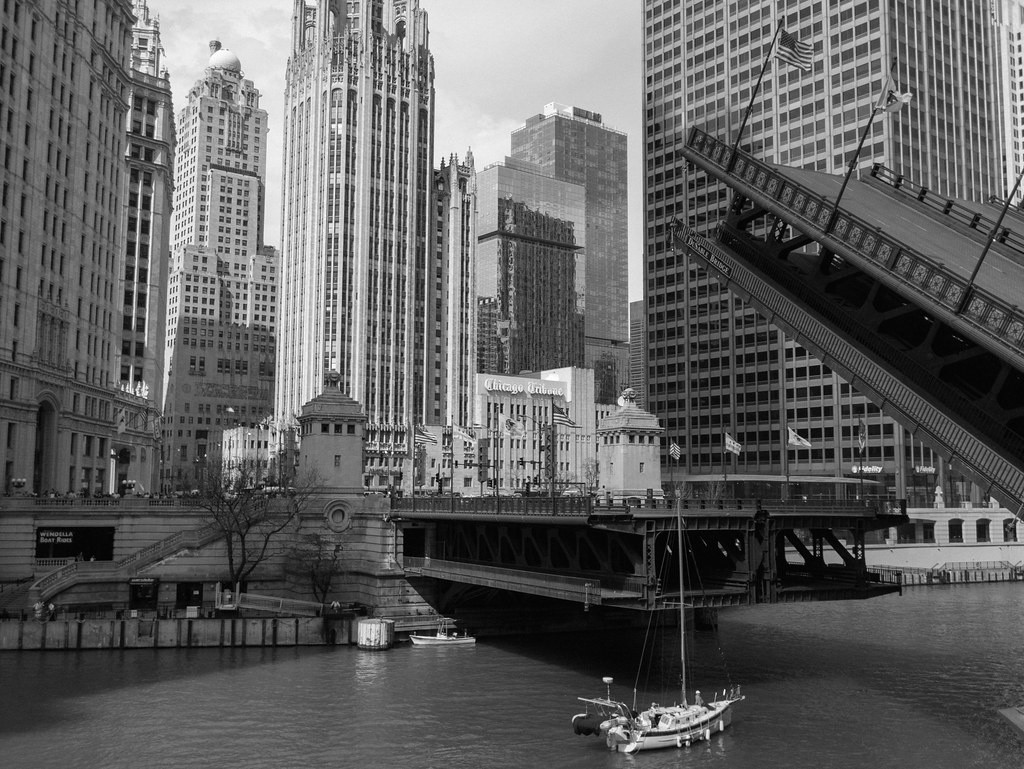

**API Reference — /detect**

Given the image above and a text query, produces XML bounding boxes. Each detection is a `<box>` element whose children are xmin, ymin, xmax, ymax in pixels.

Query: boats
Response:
<box><xmin>408</xmin><ymin>618</ymin><xmax>477</xmax><ymax>645</ymax></box>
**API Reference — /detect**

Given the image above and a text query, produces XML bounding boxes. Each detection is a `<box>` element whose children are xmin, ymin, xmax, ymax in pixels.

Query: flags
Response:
<box><xmin>769</xmin><ymin>28</ymin><xmax>815</xmax><ymax>72</ymax></box>
<box><xmin>669</xmin><ymin>442</ymin><xmax>681</xmax><ymax>460</ymax></box>
<box><xmin>874</xmin><ymin>72</ymin><xmax>914</xmax><ymax>113</ymax></box>
<box><xmin>725</xmin><ymin>432</ymin><xmax>742</xmax><ymax>456</ymax></box>
<box><xmin>787</xmin><ymin>426</ymin><xmax>813</xmax><ymax>447</ymax></box>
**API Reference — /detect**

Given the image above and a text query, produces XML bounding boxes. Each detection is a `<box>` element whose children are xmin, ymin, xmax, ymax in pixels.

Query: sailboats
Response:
<box><xmin>570</xmin><ymin>496</ymin><xmax>746</xmax><ymax>755</ymax></box>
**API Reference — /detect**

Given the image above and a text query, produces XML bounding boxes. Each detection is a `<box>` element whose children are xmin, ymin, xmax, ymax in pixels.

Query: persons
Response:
<box><xmin>0</xmin><ymin>485</ymin><xmax>297</xmax><ymax>501</ymax></box>
<box><xmin>602</xmin><ymin>485</ymin><xmax>606</xmax><ymax>493</ymax></box>
<box><xmin>694</xmin><ymin>689</ymin><xmax>716</xmax><ymax>710</ymax></box>
<box><xmin>648</xmin><ymin>701</ymin><xmax>664</xmax><ymax>729</ymax></box>
<box><xmin>33</xmin><ymin>600</ymin><xmax>59</xmax><ymax>622</ymax></box>
